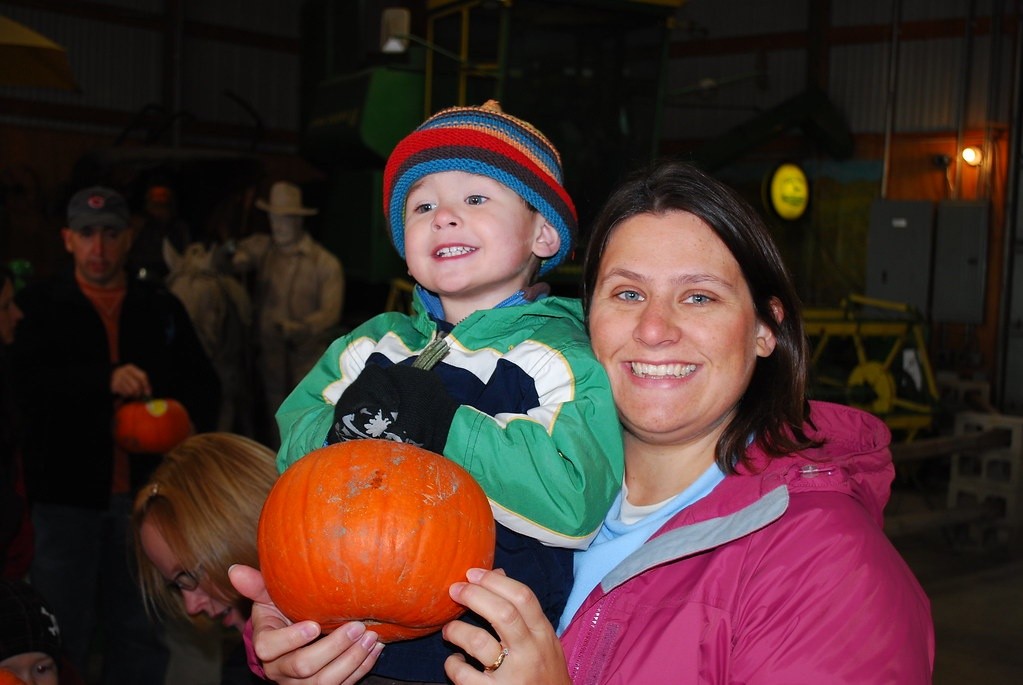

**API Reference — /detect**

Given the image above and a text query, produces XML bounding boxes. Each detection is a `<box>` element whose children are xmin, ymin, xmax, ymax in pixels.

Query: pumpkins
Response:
<box><xmin>113</xmin><ymin>398</ymin><xmax>191</xmax><ymax>454</ymax></box>
<box><xmin>255</xmin><ymin>437</ymin><xmax>495</xmax><ymax>643</ymax></box>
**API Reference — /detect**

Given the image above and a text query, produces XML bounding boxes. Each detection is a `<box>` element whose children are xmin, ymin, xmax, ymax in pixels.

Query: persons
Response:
<box><xmin>16</xmin><ymin>187</ymin><xmax>221</xmax><ymax>685</ymax></box>
<box><xmin>274</xmin><ymin>100</ymin><xmax>624</xmax><ymax>685</ymax></box>
<box><xmin>126</xmin><ymin>178</ymin><xmax>267</xmax><ymax>273</ymax></box>
<box><xmin>126</xmin><ymin>430</ymin><xmax>278</xmax><ymax>635</ymax></box>
<box><xmin>0</xmin><ymin>578</ymin><xmax>83</xmax><ymax>685</ymax></box>
<box><xmin>222</xmin><ymin>161</ymin><xmax>935</xmax><ymax>685</ymax></box>
<box><xmin>257</xmin><ymin>181</ymin><xmax>343</xmax><ymax>415</ymax></box>
<box><xmin>0</xmin><ymin>262</ymin><xmax>33</xmax><ymax>583</ymax></box>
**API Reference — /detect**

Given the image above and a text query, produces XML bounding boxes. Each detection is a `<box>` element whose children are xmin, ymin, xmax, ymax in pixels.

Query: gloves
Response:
<box><xmin>335</xmin><ymin>351</ymin><xmax>456</xmax><ymax>451</ymax></box>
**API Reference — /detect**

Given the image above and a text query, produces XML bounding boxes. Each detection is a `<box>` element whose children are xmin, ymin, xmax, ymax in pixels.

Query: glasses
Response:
<box><xmin>167</xmin><ymin>566</ymin><xmax>200</xmax><ymax>599</ymax></box>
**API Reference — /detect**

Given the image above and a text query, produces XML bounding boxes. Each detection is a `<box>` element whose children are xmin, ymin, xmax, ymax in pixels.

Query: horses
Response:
<box><xmin>161</xmin><ymin>235</ymin><xmax>253</xmax><ymax>432</ymax></box>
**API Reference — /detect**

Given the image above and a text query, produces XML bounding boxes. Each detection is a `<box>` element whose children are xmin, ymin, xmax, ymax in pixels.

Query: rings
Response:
<box><xmin>485</xmin><ymin>645</ymin><xmax>508</xmax><ymax>672</ymax></box>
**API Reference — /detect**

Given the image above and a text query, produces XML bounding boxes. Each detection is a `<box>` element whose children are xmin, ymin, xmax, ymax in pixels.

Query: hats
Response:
<box><xmin>0</xmin><ymin>579</ymin><xmax>62</xmax><ymax>665</ymax></box>
<box><xmin>65</xmin><ymin>187</ymin><xmax>130</xmax><ymax>231</ymax></box>
<box><xmin>383</xmin><ymin>98</ymin><xmax>578</xmax><ymax>280</ymax></box>
<box><xmin>256</xmin><ymin>180</ymin><xmax>319</xmax><ymax>216</ymax></box>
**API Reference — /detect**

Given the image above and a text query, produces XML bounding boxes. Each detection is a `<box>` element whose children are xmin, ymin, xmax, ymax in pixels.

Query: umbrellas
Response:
<box><xmin>0</xmin><ymin>16</ymin><xmax>64</xmax><ymax>88</ymax></box>
